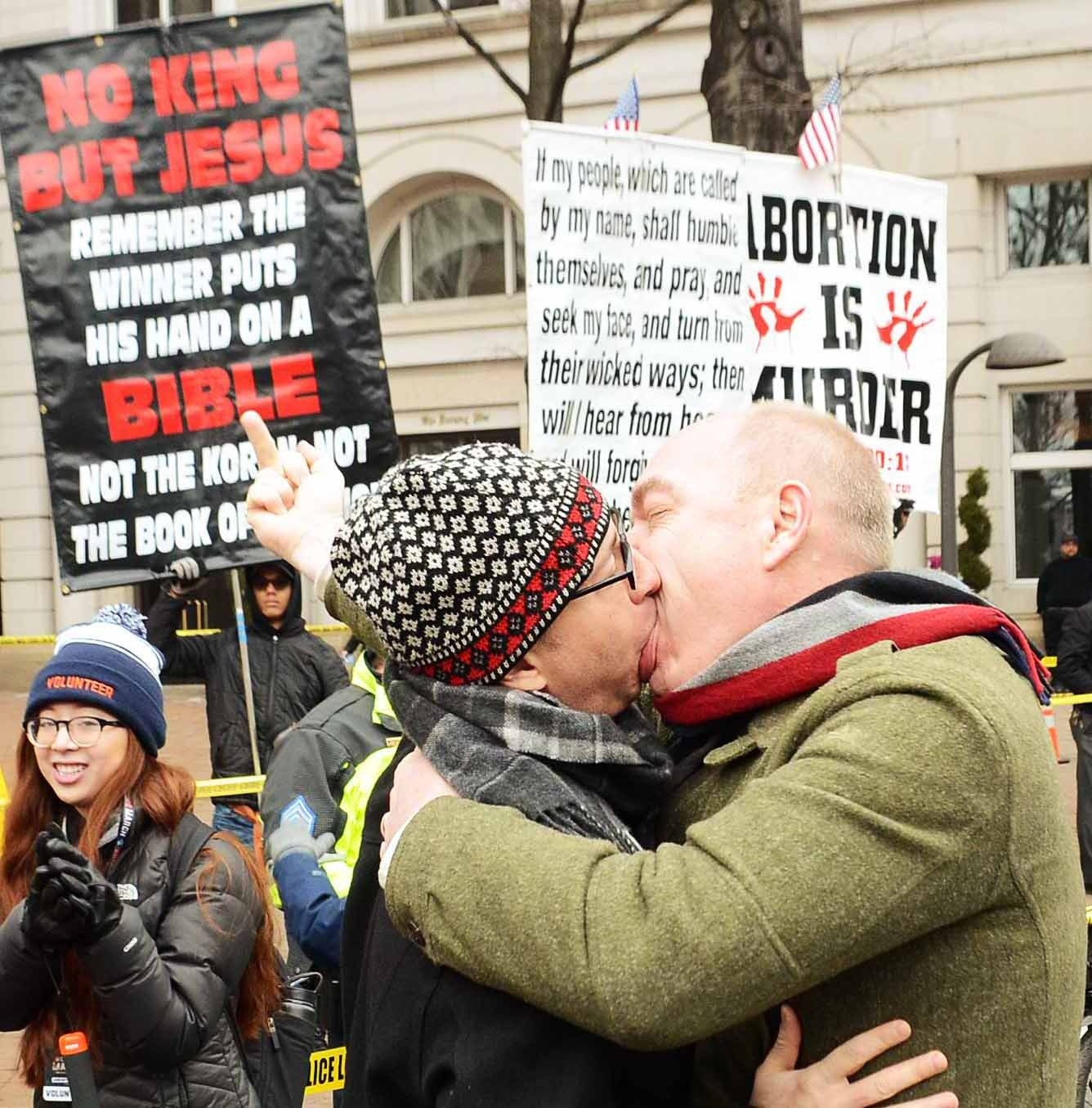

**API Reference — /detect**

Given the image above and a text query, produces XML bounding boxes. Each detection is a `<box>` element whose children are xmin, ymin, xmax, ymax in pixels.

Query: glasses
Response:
<box><xmin>23</xmin><ymin>717</ymin><xmax>128</xmax><ymax>747</ymax></box>
<box><xmin>252</xmin><ymin>574</ymin><xmax>293</xmax><ymax>591</ymax></box>
<box><xmin>570</xmin><ymin>507</ymin><xmax>635</xmax><ymax>602</ymax></box>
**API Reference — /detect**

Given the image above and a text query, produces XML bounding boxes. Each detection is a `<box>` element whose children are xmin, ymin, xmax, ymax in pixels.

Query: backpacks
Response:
<box><xmin>173</xmin><ymin>813</ymin><xmax>317</xmax><ymax>1108</ymax></box>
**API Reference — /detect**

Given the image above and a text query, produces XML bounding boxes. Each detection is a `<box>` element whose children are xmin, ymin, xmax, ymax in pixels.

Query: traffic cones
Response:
<box><xmin>1041</xmin><ymin>704</ymin><xmax>1071</xmax><ymax>764</ymax></box>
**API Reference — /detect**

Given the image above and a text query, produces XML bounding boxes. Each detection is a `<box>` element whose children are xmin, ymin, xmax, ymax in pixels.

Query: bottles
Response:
<box><xmin>279</xmin><ymin>971</ymin><xmax>324</xmax><ymax>1023</ymax></box>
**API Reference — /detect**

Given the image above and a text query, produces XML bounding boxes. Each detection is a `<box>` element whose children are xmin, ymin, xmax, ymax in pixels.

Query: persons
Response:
<box><xmin>241</xmin><ymin>399</ymin><xmax>1089</xmax><ymax>1108</ymax></box>
<box><xmin>1037</xmin><ymin>533</ymin><xmax>1092</xmax><ymax>656</ymax></box>
<box><xmin>0</xmin><ymin>603</ymin><xmax>284</xmax><ymax>1108</ymax></box>
<box><xmin>259</xmin><ymin>642</ymin><xmax>404</xmax><ymax>970</ymax></box>
<box><xmin>1056</xmin><ymin>599</ymin><xmax>1092</xmax><ymax>893</ymax></box>
<box><xmin>146</xmin><ymin>556</ymin><xmax>349</xmax><ymax>850</ymax></box>
<box><xmin>329</xmin><ymin>443</ymin><xmax>958</xmax><ymax>1108</ymax></box>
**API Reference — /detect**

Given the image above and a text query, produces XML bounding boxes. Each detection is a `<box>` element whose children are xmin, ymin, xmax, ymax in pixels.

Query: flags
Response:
<box><xmin>798</xmin><ymin>72</ymin><xmax>841</xmax><ymax>168</ymax></box>
<box><xmin>603</xmin><ymin>80</ymin><xmax>639</xmax><ymax>131</ymax></box>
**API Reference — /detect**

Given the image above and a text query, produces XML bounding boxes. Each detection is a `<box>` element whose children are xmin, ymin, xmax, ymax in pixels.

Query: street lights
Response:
<box><xmin>940</xmin><ymin>333</ymin><xmax>1067</xmax><ymax>580</ymax></box>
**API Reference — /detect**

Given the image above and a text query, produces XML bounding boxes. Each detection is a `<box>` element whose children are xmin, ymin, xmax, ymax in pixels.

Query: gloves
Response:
<box><xmin>268</xmin><ymin>825</ymin><xmax>335</xmax><ymax>864</ymax></box>
<box><xmin>168</xmin><ymin>557</ymin><xmax>211</xmax><ymax>596</ymax></box>
<box><xmin>23</xmin><ymin>821</ymin><xmax>122</xmax><ymax>952</ymax></box>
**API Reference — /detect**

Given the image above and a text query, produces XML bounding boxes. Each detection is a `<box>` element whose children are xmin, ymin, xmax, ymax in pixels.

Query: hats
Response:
<box><xmin>28</xmin><ymin>602</ymin><xmax>167</xmax><ymax>756</ymax></box>
<box><xmin>1061</xmin><ymin>536</ymin><xmax>1077</xmax><ymax>544</ymax></box>
<box><xmin>331</xmin><ymin>445</ymin><xmax>613</xmax><ymax>685</ymax></box>
